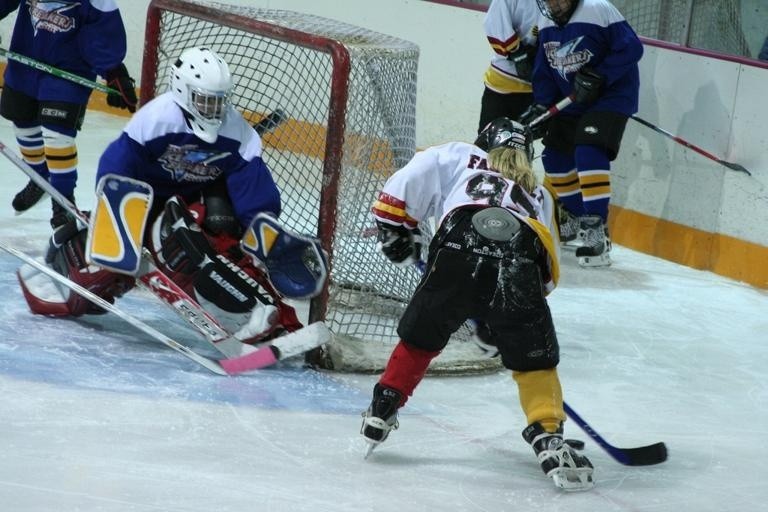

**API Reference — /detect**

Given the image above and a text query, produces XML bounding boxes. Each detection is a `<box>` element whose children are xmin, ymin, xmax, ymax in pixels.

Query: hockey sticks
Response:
<box><xmin>0</xmin><ymin>141</ymin><xmax>333</xmax><ymax>363</ymax></box>
<box><xmin>413</xmin><ymin>255</ymin><xmax>669</xmax><ymax>467</ymax></box>
<box><xmin>630</xmin><ymin>113</ymin><xmax>755</xmax><ymax>178</ymax></box>
<box><xmin>0</xmin><ymin>48</ymin><xmax>288</xmax><ymax>137</ymax></box>
<box><xmin>340</xmin><ymin>91</ymin><xmax>579</xmax><ymax>238</ymax></box>
<box><xmin>1</xmin><ymin>242</ymin><xmax>281</xmax><ymax>377</ymax></box>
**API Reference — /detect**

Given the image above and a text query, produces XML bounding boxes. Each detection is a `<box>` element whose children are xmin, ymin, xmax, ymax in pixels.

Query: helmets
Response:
<box><xmin>171</xmin><ymin>48</ymin><xmax>230</xmax><ymax>142</ymax></box>
<box><xmin>478</xmin><ymin>118</ymin><xmax>534</xmax><ymax>160</ymax></box>
<box><xmin>537</xmin><ymin>0</ymin><xmax>580</xmax><ymax>24</ymax></box>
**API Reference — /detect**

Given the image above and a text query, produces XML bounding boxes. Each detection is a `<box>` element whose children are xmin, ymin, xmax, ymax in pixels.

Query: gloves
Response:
<box><xmin>510</xmin><ymin>47</ymin><xmax>535</xmax><ymax>78</ymax></box>
<box><xmin>383</xmin><ymin>230</ymin><xmax>421</xmax><ymax>263</ymax></box>
<box><xmin>572</xmin><ymin>72</ymin><xmax>605</xmax><ymax>109</ymax></box>
<box><xmin>522</xmin><ymin>104</ymin><xmax>548</xmax><ymax>135</ymax></box>
<box><xmin>105</xmin><ymin>66</ymin><xmax>140</xmax><ymax>112</ymax></box>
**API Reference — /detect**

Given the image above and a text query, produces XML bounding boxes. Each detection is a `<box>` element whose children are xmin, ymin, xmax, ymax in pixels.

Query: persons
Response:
<box><xmin>16</xmin><ymin>46</ymin><xmax>324</xmax><ymax>369</ymax></box>
<box><xmin>360</xmin><ymin>118</ymin><xmax>597</xmax><ymax>492</ymax></box>
<box><xmin>0</xmin><ymin>0</ymin><xmax>138</xmax><ymax>232</ymax></box>
<box><xmin>517</xmin><ymin>0</ymin><xmax>644</xmax><ymax>269</ymax></box>
<box><xmin>477</xmin><ymin>0</ymin><xmax>541</xmax><ymax>136</ymax></box>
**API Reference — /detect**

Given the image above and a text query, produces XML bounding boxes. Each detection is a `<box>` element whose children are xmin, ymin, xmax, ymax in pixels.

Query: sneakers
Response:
<box><xmin>49</xmin><ymin>190</ymin><xmax>77</xmax><ymax>229</ymax></box>
<box><xmin>523</xmin><ymin>421</ymin><xmax>593</xmax><ymax>477</ymax></box>
<box><xmin>558</xmin><ymin>215</ymin><xmax>585</xmax><ymax>242</ymax></box>
<box><xmin>362</xmin><ymin>384</ymin><xmax>403</xmax><ymax>442</ymax></box>
<box><xmin>12</xmin><ymin>177</ymin><xmax>50</xmax><ymax>211</ymax></box>
<box><xmin>575</xmin><ymin>216</ymin><xmax>611</xmax><ymax>255</ymax></box>
